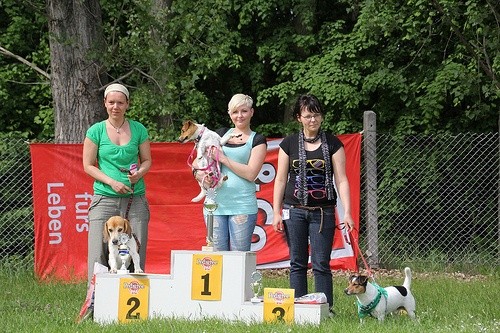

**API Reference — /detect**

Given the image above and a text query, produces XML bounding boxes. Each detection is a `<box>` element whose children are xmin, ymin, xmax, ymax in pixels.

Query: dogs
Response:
<box><xmin>342</xmin><ymin>265</ymin><xmax>417</xmax><ymax>326</ymax></box>
<box><xmin>101</xmin><ymin>214</ymin><xmax>144</xmax><ymax>274</ymax></box>
<box><xmin>176</xmin><ymin>117</ymin><xmax>222</xmax><ymax>203</ymax></box>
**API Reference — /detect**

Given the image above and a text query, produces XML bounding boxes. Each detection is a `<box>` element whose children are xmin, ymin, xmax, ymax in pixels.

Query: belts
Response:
<box><xmin>296</xmin><ymin>205</ymin><xmax>332</xmax><ymax>234</ymax></box>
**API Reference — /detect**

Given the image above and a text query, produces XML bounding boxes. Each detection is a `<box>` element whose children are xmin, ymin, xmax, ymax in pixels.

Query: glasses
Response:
<box><xmin>300</xmin><ymin>113</ymin><xmax>321</xmax><ymax>120</ymax></box>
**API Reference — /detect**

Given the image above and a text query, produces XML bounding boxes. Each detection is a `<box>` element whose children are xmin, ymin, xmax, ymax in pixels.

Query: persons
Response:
<box><xmin>273</xmin><ymin>95</ymin><xmax>354</xmax><ymax>317</ymax></box>
<box><xmin>193</xmin><ymin>94</ymin><xmax>268</xmax><ymax>251</ymax></box>
<box><xmin>83</xmin><ymin>82</ymin><xmax>151</xmax><ymax>319</ymax></box>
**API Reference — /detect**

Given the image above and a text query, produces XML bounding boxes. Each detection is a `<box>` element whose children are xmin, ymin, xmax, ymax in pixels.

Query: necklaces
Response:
<box><xmin>232</xmin><ymin>126</ymin><xmax>249</xmax><ymax>140</ymax></box>
<box><xmin>108</xmin><ymin>116</ymin><xmax>125</xmax><ymax>132</ymax></box>
